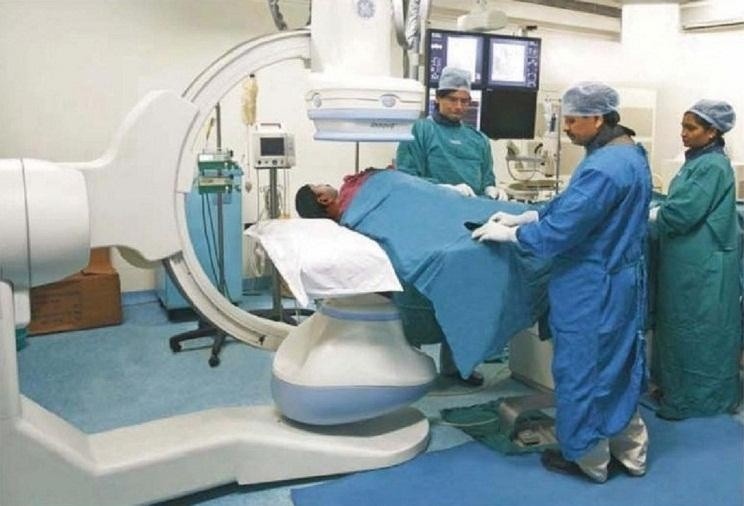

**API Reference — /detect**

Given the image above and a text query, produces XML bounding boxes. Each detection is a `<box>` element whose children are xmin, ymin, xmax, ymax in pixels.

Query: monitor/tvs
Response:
<box><xmin>253</xmin><ymin>130</ymin><xmax>300</xmax><ymax>170</ymax></box>
<box><xmin>483</xmin><ymin>85</ymin><xmax>538</xmax><ymax>140</ymax></box>
<box><xmin>424</xmin><ymin>86</ymin><xmax>484</xmax><ymax>132</ymax></box>
<box><xmin>425</xmin><ymin>27</ymin><xmax>487</xmax><ymax>88</ymax></box>
<box><xmin>487</xmin><ymin>33</ymin><xmax>542</xmax><ymax>91</ymax></box>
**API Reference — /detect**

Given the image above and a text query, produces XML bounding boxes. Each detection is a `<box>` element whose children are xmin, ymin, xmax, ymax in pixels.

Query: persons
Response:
<box><xmin>472</xmin><ymin>84</ymin><xmax>652</xmax><ymax>483</ymax></box>
<box><xmin>647</xmin><ymin>99</ymin><xmax>744</xmax><ymax>421</ymax></box>
<box><xmin>296</xmin><ymin>167</ymin><xmax>382</xmax><ymax>223</ymax></box>
<box><xmin>398</xmin><ymin>68</ymin><xmax>511</xmax><ymax>202</ymax></box>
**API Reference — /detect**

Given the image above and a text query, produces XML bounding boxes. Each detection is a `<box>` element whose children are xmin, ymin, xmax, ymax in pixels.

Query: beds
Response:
<box><xmin>242</xmin><ymin>218</ymin><xmax>652</xmax><ymax>427</ymax></box>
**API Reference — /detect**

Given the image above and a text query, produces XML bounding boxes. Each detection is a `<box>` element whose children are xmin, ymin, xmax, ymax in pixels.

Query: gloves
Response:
<box><xmin>472</xmin><ymin>223</ymin><xmax>518</xmax><ymax>243</ymax></box>
<box><xmin>490</xmin><ymin>211</ymin><xmax>540</xmax><ymax>227</ymax></box>
<box><xmin>485</xmin><ymin>186</ymin><xmax>508</xmax><ymax>201</ymax></box>
<box><xmin>437</xmin><ymin>184</ymin><xmax>476</xmax><ymax>198</ymax></box>
<box><xmin>648</xmin><ymin>206</ymin><xmax>661</xmax><ymax>221</ymax></box>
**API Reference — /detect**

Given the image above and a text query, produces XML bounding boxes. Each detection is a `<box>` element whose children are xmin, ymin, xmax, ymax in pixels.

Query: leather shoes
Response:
<box><xmin>615</xmin><ymin>458</ymin><xmax>628</xmax><ymax>471</ymax></box>
<box><xmin>441</xmin><ymin>370</ymin><xmax>484</xmax><ymax>385</ymax></box>
<box><xmin>540</xmin><ymin>449</ymin><xmax>613</xmax><ymax>476</ymax></box>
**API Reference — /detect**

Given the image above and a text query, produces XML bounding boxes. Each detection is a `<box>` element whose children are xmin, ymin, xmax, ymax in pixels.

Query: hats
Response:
<box><xmin>562</xmin><ymin>81</ymin><xmax>621</xmax><ymax>117</ymax></box>
<box><xmin>436</xmin><ymin>67</ymin><xmax>470</xmax><ymax>91</ymax></box>
<box><xmin>687</xmin><ymin>99</ymin><xmax>735</xmax><ymax>133</ymax></box>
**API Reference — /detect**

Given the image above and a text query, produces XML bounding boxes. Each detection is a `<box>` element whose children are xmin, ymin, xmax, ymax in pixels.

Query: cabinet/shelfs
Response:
<box><xmin>521</xmin><ymin>82</ymin><xmax>657</xmax><ymax>180</ymax></box>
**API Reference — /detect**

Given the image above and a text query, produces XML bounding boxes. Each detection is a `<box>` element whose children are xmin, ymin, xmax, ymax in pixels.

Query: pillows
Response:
<box><xmin>242</xmin><ymin>219</ymin><xmax>404</xmax><ymax>311</ymax></box>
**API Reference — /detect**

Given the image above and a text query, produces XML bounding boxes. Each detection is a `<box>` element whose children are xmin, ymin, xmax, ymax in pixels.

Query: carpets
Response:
<box><xmin>18</xmin><ymin>278</ymin><xmax>533</xmax><ymax>506</ymax></box>
<box><xmin>291</xmin><ymin>393</ymin><xmax>743</xmax><ymax>505</ymax></box>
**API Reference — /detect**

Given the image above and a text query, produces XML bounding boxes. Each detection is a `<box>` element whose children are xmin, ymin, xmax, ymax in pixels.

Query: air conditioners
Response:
<box><xmin>679</xmin><ymin>1</ymin><xmax>743</xmax><ymax>30</ymax></box>
